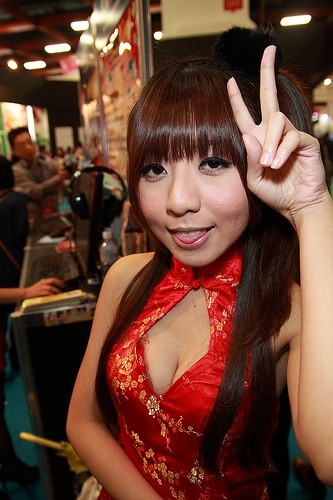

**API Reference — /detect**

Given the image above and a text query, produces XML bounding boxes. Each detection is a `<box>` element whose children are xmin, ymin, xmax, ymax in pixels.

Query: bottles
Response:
<box><xmin>100</xmin><ymin>231</ymin><xmax>116</xmax><ymax>283</ymax></box>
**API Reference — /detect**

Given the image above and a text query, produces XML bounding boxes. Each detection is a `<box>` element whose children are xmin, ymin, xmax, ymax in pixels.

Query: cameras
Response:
<box><xmin>72</xmin><ymin>169</ymin><xmax>102</xmax><ymax>276</ymax></box>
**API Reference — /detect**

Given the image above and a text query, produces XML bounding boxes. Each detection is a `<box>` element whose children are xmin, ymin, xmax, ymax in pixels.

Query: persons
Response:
<box><xmin>66</xmin><ymin>27</ymin><xmax>333</xmax><ymax>500</ymax></box>
<box><xmin>8</xmin><ymin>126</ymin><xmax>67</xmax><ymax>381</ymax></box>
<box><xmin>0</xmin><ymin>155</ymin><xmax>29</xmax><ymax>386</ymax></box>
<box><xmin>28</xmin><ymin>135</ymin><xmax>103</xmax><ymax>178</ymax></box>
<box><xmin>1</xmin><ymin>278</ymin><xmax>66</xmax><ymax>497</ymax></box>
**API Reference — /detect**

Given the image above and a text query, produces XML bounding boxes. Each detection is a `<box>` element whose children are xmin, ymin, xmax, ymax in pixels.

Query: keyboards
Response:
<box><xmin>29</xmin><ymin>251</ymin><xmax>81</xmax><ymax>287</ymax></box>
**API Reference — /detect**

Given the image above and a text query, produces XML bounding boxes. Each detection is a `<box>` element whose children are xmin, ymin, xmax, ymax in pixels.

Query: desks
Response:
<box><xmin>9</xmin><ymin>215</ymin><xmax>96</xmax><ymax>500</ymax></box>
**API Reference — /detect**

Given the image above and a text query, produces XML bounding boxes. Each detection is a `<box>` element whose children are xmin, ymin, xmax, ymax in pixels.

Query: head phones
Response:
<box><xmin>69</xmin><ymin>166</ymin><xmax>128</xmax><ymax>218</ymax></box>
